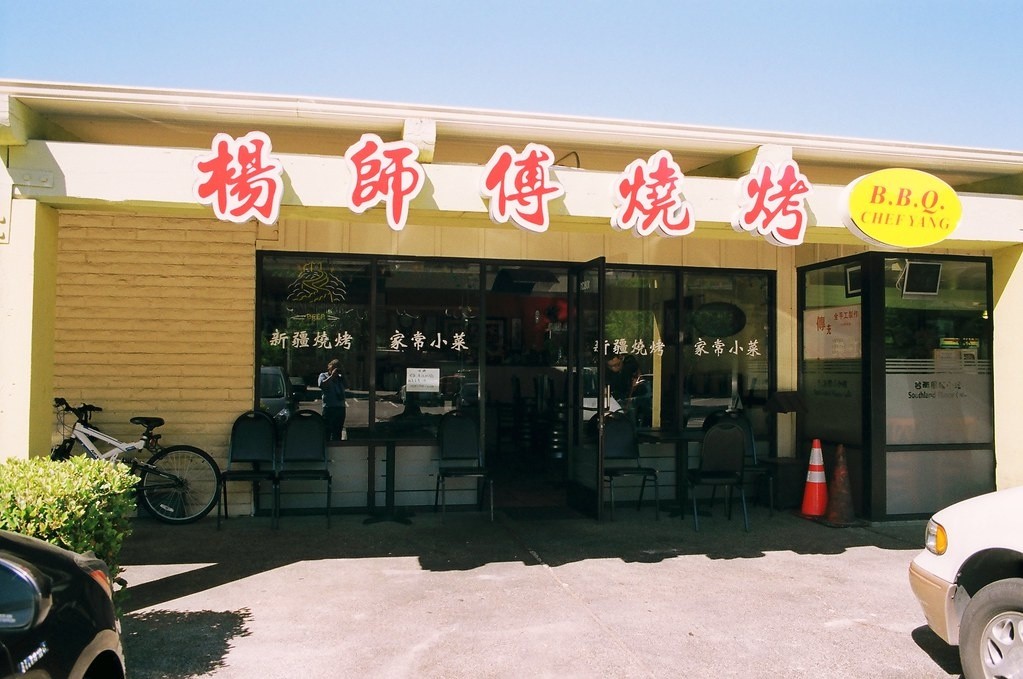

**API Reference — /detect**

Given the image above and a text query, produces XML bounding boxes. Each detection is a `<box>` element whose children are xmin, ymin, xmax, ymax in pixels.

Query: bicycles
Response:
<box><xmin>48</xmin><ymin>398</ymin><xmax>221</xmax><ymax>523</ymax></box>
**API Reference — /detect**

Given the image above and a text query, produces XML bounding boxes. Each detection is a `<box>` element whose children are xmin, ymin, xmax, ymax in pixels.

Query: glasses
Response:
<box><xmin>606</xmin><ymin>359</ymin><xmax>621</xmax><ymax>369</ymax></box>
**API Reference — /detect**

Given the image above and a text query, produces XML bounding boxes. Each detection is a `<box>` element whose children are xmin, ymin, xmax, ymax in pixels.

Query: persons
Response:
<box><xmin>605</xmin><ymin>345</ymin><xmax>640</xmax><ymax>401</ymax></box>
<box><xmin>318</xmin><ymin>359</ymin><xmax>346</xmax><ymax>439</ymax></box>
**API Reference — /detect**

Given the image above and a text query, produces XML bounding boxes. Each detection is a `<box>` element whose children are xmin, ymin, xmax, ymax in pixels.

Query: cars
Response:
<box><xmin>261</xmin><ymin>365</ymin><xmax>297</xmax><ymax>422</ymax></box>
<box><xmin>401</xmin><ymin>375</ymin><xmax>465</xmax><ymax>399</ymax></box>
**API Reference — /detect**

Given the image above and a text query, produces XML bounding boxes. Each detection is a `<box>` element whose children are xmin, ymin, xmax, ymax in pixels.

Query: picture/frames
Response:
<box><xmin>437</xmin><ymin>316</ymin><xmax>523</xmax><ymax>359</ymax></box>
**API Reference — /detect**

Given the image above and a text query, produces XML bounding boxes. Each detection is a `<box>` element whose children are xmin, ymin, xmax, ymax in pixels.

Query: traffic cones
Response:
<box><xmin>793</xmin><ymin>439</ymin><xmax>830</xmax><ymax>519</ymax></box>
<box><xmin>816</xmin><ymin>444</ymin><xmax>867</xmax><ymax>529</ymax></box>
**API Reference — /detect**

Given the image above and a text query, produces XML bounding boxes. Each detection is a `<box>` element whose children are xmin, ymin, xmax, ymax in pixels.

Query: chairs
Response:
<box><xmin>433</xmin><ymin>411</ymin><xmax>495</xmax><ymax>525</ymax></box>
<box><xmin>684</xmin><ymin>413</ymin><xmax>775</xmax><ymax>533</ymax></box>
<box><xmin>216</xmin><ymin>409</ymin><xmax>333</xmax><ymax>529</ymax></box>
<box><xmin>588</xmin><ymin>411</ymin><xmax>662</xmax><ymax>521</ymax></box>
